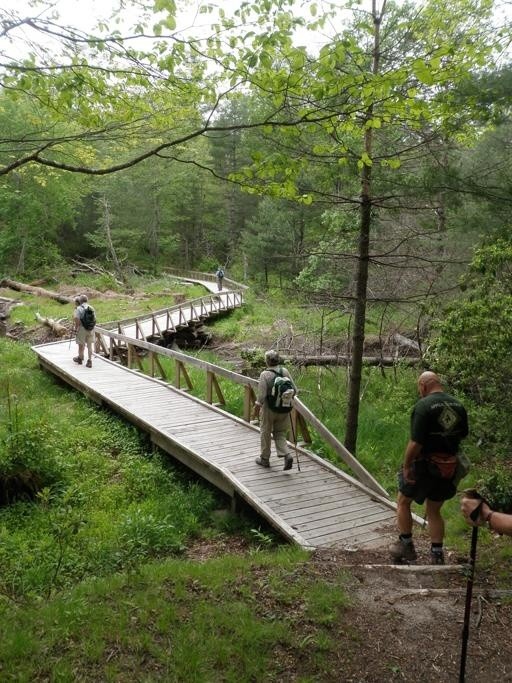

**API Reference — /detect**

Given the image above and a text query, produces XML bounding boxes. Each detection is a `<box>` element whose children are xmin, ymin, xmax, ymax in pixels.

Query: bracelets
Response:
<box><xmin>485</xmin><ymin>512</ymin><xmax>496</xmax><ymax>535</ymax></box>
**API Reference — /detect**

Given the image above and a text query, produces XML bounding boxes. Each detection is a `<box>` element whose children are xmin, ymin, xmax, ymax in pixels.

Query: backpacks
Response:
<box><xmin>219</xmin><ymin>271</ymin><xmax>223</xmax><ymax>278</ymax></box>
<box><xmin>80</xmin><ymin>306</ymin><xmax>95</xmax><ymax>330</ymax></box>
<box><xmin>266</xmin><ymin>368</ymin><xmax>295</xmax><ymax>414</ymax></box>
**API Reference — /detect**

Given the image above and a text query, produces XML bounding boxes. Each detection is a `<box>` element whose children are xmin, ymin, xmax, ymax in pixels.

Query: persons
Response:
<box><xmin>216</xmin><ymin>268</ymin><xmax>224</xmax><ymax>292</ymax></box>
<box><xmin>460</xmin><ymin>494</ymin><xmax>512</xmax><ymax>536</ymax></box>
<box><xmin>72</xmin><ymin>294</ymin><xmax>97</xmax><ymax>368</ymax></box>
<box><xmin>255</xmin><ymin>350</ymin><xmax>298</xmax><ymax>471</ymax></box>
<box><xmin>73</xmin><ymin>296</ymin><xmax>81</xmax><ymax>323</ymax></box>
<box><xmin>386</xmin><ymin>370</ymin><xmax>469</xmax><ymax>566</ymax></box>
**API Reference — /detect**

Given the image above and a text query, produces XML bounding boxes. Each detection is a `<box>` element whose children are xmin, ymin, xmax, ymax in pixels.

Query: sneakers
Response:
<box><xmin>255</xmin><ymin>457</ymin><xmax>270</xmax><ymax>467</ymax></box>
<box><xmin>426</xmin><ymin>552</ymin><xmax>445</xmax><ymax>565</ymax></box>
<box><xmin>86</xmin><ymin>360</ymin><xmax>92</xmax><ymax>368</ymax></box>
<box><xmin>388</xmin><ymin>541</ymin><xmax>417</xmax><ymax>560</ymax></box>
<box><xmin>283</xmin><ymin>454</ymin><xmax>293</xmax><ymax>470</ymax></box>
<box><xmin>73</xmin><ymin>357</ymin><xmax>82</xmax><ymax>365</ymax></box>
